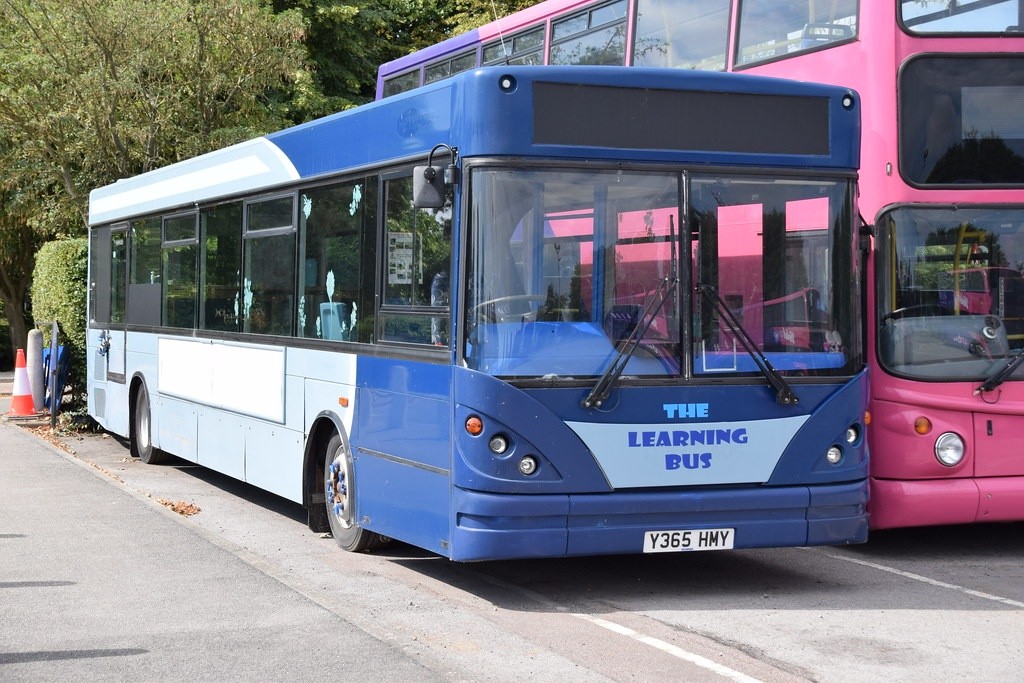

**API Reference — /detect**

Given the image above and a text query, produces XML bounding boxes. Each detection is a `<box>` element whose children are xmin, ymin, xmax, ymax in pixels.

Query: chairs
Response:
<box><xmin>167</xmin><ymin>292</ymin><xmax>360</xmax><ymax>344</ymax></box>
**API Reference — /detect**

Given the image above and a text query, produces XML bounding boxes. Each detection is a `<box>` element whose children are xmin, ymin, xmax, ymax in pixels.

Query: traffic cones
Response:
<box><xmin>7</xmin><ymin>349</ymin><xmax>43</xmax><ymax>420</ymax></box>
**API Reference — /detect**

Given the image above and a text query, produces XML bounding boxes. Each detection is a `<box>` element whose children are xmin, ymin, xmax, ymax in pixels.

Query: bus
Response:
<box><xmin>86</xmin><ymin>2</ymin><xmax>866</xmax><ymax>564</ymax></box>
<box><xmin>376</xmin><ymin>2</ymin><xmax>1024</xmax><ymax>534</ymax></box>
